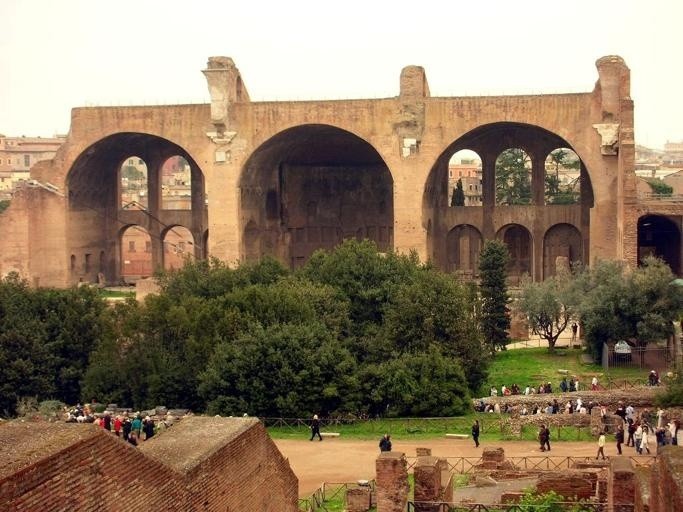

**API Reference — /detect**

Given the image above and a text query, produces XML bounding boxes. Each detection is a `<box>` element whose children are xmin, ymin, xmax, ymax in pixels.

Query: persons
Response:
<box><xmin>472</xmin><ymin>369</ymin><xmax>680</xmax><ymax>460</ymax></box>
<box><xmin>378</xmin><ymin>431</ymin><xmax>391</xmax><ymax>452</ymax></box>
<box><xmin>62</xmin><ymin>397</ymin><xmax>176</xmax><ymax>448</ymax></box>
<box><xmin>308</xmin><ymin>413</ymin><xmax>322</xmax><ymax>441</ymax></box>
